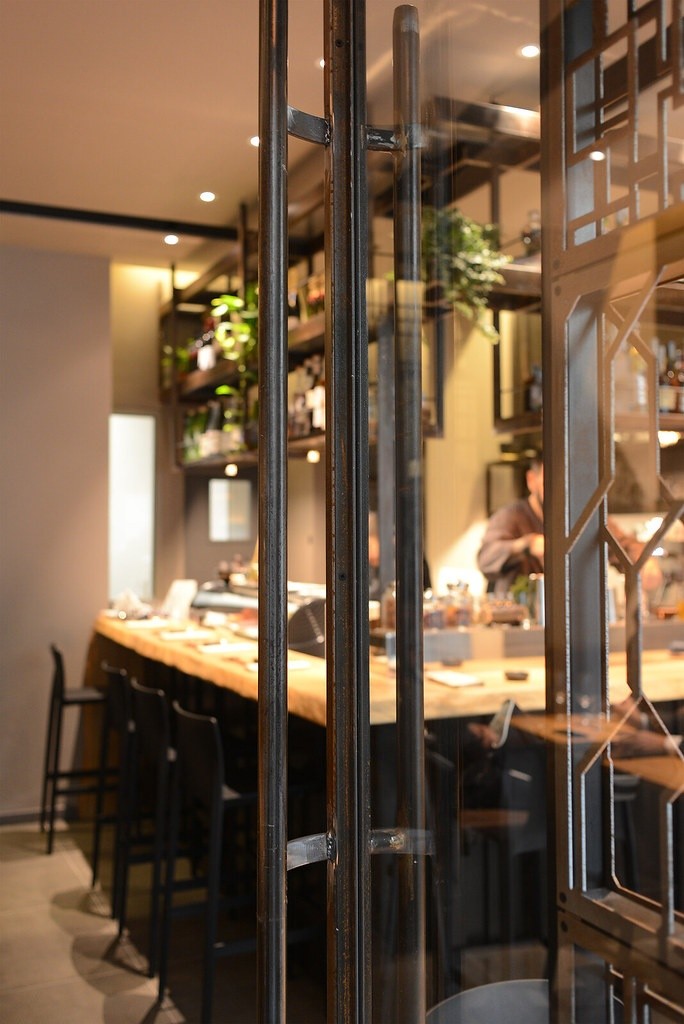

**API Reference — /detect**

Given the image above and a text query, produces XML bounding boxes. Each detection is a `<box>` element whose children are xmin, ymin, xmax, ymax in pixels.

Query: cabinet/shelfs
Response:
<box><xmin>158</xmin><ymin>161</ymin><xmax>449</xmax><ymax>476</ymax></box>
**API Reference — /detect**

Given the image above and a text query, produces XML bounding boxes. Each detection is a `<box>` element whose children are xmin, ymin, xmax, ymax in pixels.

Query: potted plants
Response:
<box><xmin>427</xmin><ymin>200</ymin><xmax>515</xmax><ymax>352</ymax></box>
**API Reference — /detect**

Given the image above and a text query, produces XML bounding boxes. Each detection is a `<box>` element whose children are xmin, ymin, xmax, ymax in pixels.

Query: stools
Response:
<box><xmin>38</xmin><ymin>643</ymin><xmax>330</xmax><ymax>1024</ymax></box>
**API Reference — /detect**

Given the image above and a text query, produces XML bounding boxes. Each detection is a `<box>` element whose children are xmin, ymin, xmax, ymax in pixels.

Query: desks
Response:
<box><xmin>92</xmin><ymin>606</ymin><xmax>684</xmax><ymax>972</ymax></box>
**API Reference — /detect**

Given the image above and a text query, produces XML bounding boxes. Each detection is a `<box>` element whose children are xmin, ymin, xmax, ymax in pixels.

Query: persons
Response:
<box><xmin>478</xmin><ymin>451</ymin><xmax>544</xmax><ymax>603</ymax></box>
<box><xmin>368</xmin><ymin>512</ymin><xmax>432</xmax><ymax>600</ymax></box>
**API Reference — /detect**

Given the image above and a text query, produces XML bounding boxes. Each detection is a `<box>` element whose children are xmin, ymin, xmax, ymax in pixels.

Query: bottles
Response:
<box><xmin>160</xmin><ymin>268</ymin><xmax>327</xmax><ymax>460</ymax></box>
<box><xmin>379</xmin><ymin>581</ymin><xmax>475</xmax><ymax>632</ymax></box>
